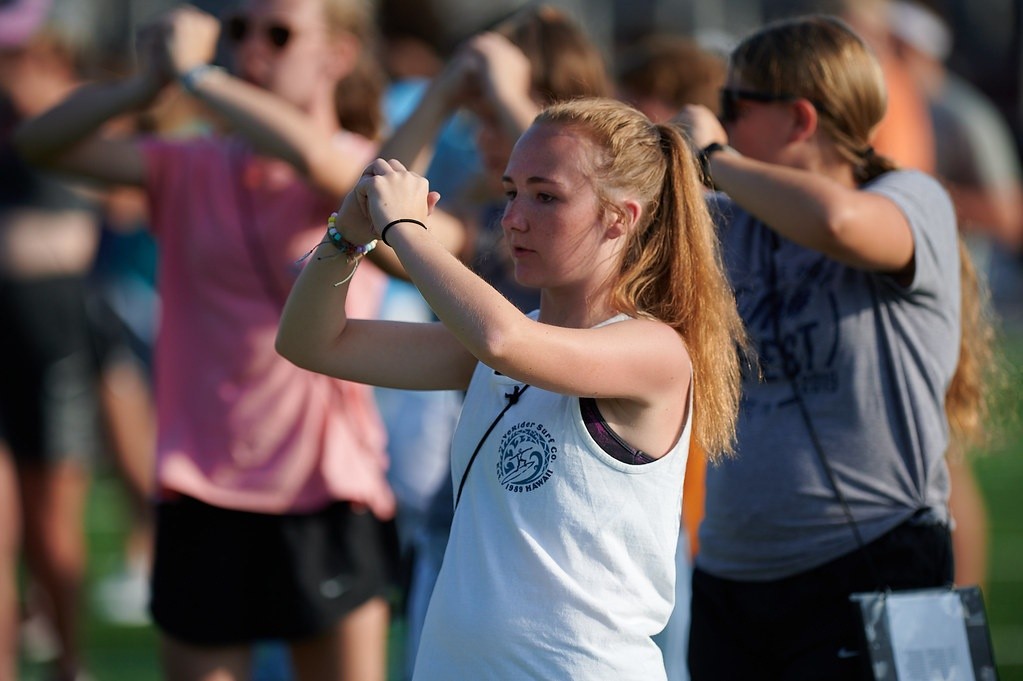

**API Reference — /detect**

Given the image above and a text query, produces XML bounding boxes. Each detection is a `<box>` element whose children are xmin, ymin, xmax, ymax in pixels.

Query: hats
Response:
<box><xmin>887</xmin><ymin>1</ymin><xmax>951</xmax><ymax>63</ymax></box>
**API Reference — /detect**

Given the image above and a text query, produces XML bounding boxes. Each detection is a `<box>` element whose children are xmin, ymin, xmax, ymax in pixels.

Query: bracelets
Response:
<box><xmin>294</xmin><ymin>217</ymin><xmax>378</xmax><ymax>286</ymax></box>
<box><xmin>381</xmin><ymin>219</ymin><xmax>428</xmax><ymax>247</ymax></box>
<box><xmin>182</xmin><ymin>65</ymin><xmax>214</xmax><ymax>89</ymax></box>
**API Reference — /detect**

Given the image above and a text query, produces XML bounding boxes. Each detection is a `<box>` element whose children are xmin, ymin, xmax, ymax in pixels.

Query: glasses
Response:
<box><xmin>721</xmin><ymin>85</ymin><xmax>827</xmax><ymax>123</ymax></box>
<box><xmin>227</xmin><ymin>16</ymin><xmax>291</xmax><ymax>49</ymax></box>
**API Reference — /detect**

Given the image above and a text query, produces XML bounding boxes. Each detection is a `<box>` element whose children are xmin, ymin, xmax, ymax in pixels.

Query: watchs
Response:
<box><xmin>699</xmin><ymin>144</ymin><xmax>739</xmax><ymax>191</ymax></box>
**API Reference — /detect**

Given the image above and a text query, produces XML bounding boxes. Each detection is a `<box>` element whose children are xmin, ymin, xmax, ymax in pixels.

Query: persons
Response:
<box><xmin>274</xmin><ymin>99</ymin><xmax>759</xmax><ymax>681</ymax></box>
<box><xmin>0</xmin><ymin>1</ymin><xmax>1023</xmax><ymax>680</ymax></box>
<box><xmin>19</xmin><ymin>0</ymin><xmax>460</xmax><ymax>681</ymax></box>
<box><xmin>677</xmin><ymin>16</ymin><xmax>956</xmax><ymax>681</ymax></box>
